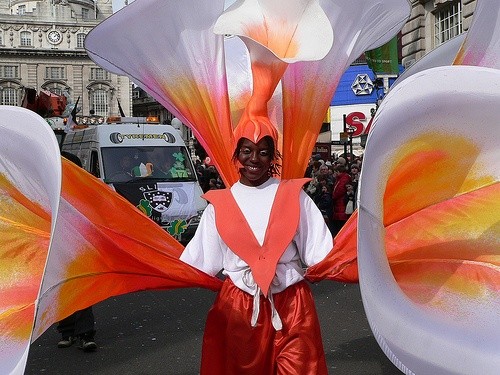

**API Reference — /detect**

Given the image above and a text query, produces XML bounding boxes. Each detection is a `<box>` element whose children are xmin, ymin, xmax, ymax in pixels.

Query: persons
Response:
<box><xmin>179</xmin><ymin>116</ymin><xmax>357</xmax><ymax>375</ymax></box>
<box><xmin>114</xmin><ymin>154</ymin><xmax>135</xmax><ymax>182</ymax></box>
<box><xmin>303</xmin><ymin>153</ymin><xmax>364</xmax><ymax>237</ymax></box>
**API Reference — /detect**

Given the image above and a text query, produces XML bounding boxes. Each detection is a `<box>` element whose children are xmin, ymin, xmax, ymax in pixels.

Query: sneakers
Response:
<box><xmin>58</xmin><ymin>330</ymin><xmax>78</xmax><ymax>347</ymax></box>
<box><xmin>77</xmin><ymin>329</ymin><xmax>98</xmax><ymax>351</ymax></box>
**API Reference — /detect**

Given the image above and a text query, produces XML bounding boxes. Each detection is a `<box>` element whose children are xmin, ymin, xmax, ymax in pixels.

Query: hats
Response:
<box><xmin>351</xmin><ymin>164</ymin><xmax>360</xmax><ymax>172</ymax></box>
<box><xmin>214</xmin><ymin>0</ymin><xmax>334</xmax><ymax>169</ymax></box>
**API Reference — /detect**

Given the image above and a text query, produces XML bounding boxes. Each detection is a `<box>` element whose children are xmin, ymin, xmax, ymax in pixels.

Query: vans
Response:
<box><xmin>60</xmin><ymin>116</ymin><xmax>209</xmax><ymax>249</ymax></box>
